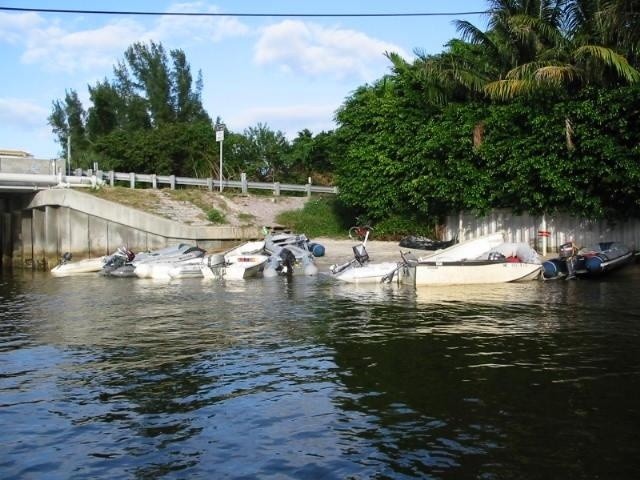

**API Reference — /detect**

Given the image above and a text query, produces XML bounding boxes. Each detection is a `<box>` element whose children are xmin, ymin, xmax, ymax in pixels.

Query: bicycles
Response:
<box><xmin>349</xmin><ymin>216</ymin><xmax>374</xmax><ymax>240</ymax></box>
